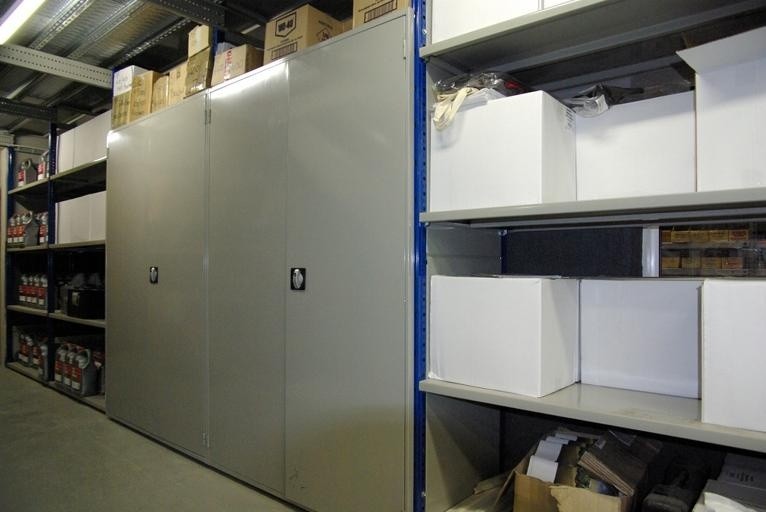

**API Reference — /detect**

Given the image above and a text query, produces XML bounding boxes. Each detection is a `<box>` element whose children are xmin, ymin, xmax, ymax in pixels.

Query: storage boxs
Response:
<box><xmin>642</xmin><ymin>462</ymin><xmax>700</xmax><ymax>512</ymax></box>
<box><xmin>674</xmin><ymin>25</ymin><xmax>766</xmax><ymax>193</ymax></box>
<box><xmin>605</xmin><ymin>429</ymin><xmax>672</xmax><ymax>471</ymax></box>
<box><xmin>489</xmin><ymin>421</ymin><xmax>649</xmax><ymax>512</ymax></box>
<box><xmin>55</xmin><ymin>190</ymin><xmax>107</xmax><ymax>245</ymax></box>
<box><xmin>572</xmin><ymin>88</ymin><xmax>696</xmax><ymax>201</ymax></box>
<box><xmin>700</xmin><ymin>276</ymin><xmax>766</xmax><ymax>433</ymax></box>
<box><xmin>57</xmin><ymin>109</ymin><xmax>112</xmax><ymax>174</ymax></box>
<box><xmin>579</xmin><ymin>278</ymin><xmax>701</xmax><ymax>399</ymax></box>
<box><xmin>716</xmin><ymin>452</ymin><xmax>766</xmax><ymax>491</ymax></box>
<box><xmin>429</xmin><ymin>90</ymin><xmax>577</xmax><ymax>213</ymax></box>
<box><xmin>691</xmin><ymin>479</ymin><xmax>766</xmax><ymax>512</ymax></box>
<box><xmin>427</xmin><ymin>274</ymin><xmax>580</xmax><ymax>399</ymax></box>
<box><xmin>431</xmin><ymin>0</ymin><xmax>538</xmax><ymax>45</ymax></box>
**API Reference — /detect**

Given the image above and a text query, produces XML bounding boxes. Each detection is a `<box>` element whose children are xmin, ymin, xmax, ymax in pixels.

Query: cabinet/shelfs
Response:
<box><xmin>414</xmin><ymin>0</ymin><xmax>765</xmax><ymax>512</ymax></box>
<box><xmin>0</xmin><ymin>149</ymin><xmax>107</xmax><ymax>416</ymax></box>
